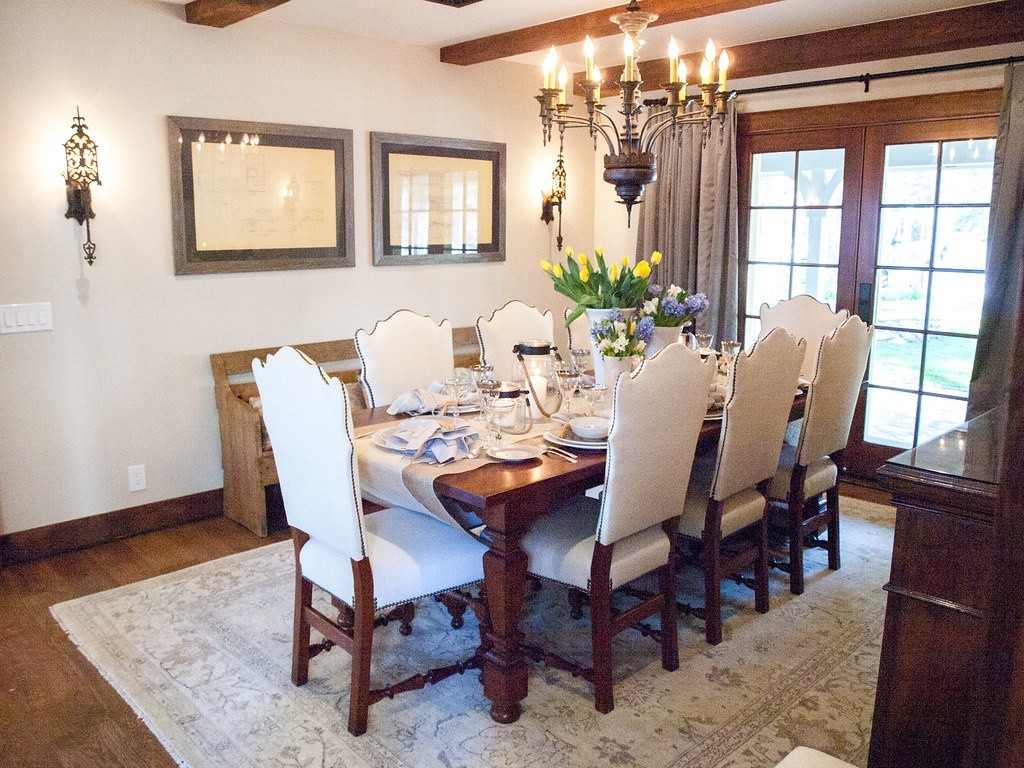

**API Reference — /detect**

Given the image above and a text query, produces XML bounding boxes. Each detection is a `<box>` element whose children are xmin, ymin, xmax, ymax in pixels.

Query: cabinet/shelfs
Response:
<box><xmin>868</xmin><ymin>403</ymin><xmax>1009</xmax><ymax>768</ymax></box>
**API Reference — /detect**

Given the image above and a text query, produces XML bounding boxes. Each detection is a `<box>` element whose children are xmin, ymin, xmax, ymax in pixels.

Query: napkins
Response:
<box><xmin>385</xmin><ymin>420</ymin><xmax>486</xmax><ymax>468</ymax></box>
<box><xmin>385</xmin><ymin>381</ymin><xmax>451</xmax><ymax>418</ymax></box>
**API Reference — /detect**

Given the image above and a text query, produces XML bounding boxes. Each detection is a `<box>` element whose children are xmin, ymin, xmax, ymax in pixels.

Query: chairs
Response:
<box><xmin>599</xmin><ymin>325</ymin><xmax>808</xmax><ymax>644</ymax></box>
<box><xmin>350</xmin><ymin>295</ymin><xmax>848</xmax><ymax>531</ymax></box>
<box><xmin>352</xmin><ymin>301</ymin><xmax>600</xmax><ymax>530</ymax></box>
<box><xmin>480</xmin><ymin>341</ymin><xmax>718</xmax><ymax>715</ymax></box>
<box><xmin>703</xmin><ymin>316</ymin><xmax>876</xmax><ymax>593</ymax></box>
<box><xmin>252</xmin><ymin>345</ymin><xmax>487</xmax><ymax>737</ymax></box>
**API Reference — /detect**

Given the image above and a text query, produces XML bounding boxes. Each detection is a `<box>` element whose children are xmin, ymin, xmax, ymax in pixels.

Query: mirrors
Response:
<box><xmin>369</xmin><ymin>131</ymin><xmax>507</xmax><ymax>267</ymax></box>
<box><xmin>164</xmin><ymin>114</ymin><xmax>355</xmax><ymax>268</ymax></box>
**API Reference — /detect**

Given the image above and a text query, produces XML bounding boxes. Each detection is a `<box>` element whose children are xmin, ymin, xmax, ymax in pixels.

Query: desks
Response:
<box><xmin>347</xmin><ymin>372</ymin><xmax>868</xmax><ymax>725</ymax></box>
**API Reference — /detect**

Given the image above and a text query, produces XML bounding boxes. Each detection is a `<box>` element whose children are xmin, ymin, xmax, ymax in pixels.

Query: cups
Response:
<box><xmin>707</xmin><ymin>397</ymin><xmax>715</xmax><ymax>410</ymax></box>
<box><xmin>695</xmin><ymin>333</ymin><xmax>714</xmax><ymax>350</ymax></box>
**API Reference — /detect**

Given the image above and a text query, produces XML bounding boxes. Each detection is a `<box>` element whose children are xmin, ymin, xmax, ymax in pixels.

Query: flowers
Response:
<box><xmin>538</xmin><ymin>245</ymin><xmax>710</xmax><ymax>363</ymax></box>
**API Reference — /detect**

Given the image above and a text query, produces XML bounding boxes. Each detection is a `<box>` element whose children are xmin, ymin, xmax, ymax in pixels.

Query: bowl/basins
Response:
<box><xmin>570</xmin><ymin>416</ymin><xmax>611</xmax><ymax>442</ymax></box>
<box><xmin>399</xmin><ymin>418</ymin><xmax>438</xmax><ymax>430</ymax></box>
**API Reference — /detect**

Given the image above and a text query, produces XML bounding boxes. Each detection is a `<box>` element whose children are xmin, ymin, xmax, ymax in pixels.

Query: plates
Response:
<box><xmin>373</xmin><ymin>425</ymin><xmax>431</xmax><ymax>453</ymax></box>
<box><xmin>543</xmin><ymin>428</ymin><xmax>609</xmax><ymax>450</ymax></box>
<box><xmin>432</xmin><ymin>404</ymin><xmax>481</xmax><ymax>413</ymax></box>
<box><xmin>487</xmin><ymin>444</ymin><xmax>546</xmax><ymax>460</ymax></box>
<box><xmin>703</xmin><ymin>410</ymin><xmax>724</xmax><ymax>421</ymax></box>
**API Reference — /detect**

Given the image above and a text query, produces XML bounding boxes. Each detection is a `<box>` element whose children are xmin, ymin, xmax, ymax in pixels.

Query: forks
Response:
<box><xmin>534</xmin><ymin>437</ymin><xmax>578</xmax><ymax>465</ymax></box>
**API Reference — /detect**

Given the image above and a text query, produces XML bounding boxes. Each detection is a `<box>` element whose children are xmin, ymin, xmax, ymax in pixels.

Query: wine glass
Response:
<box><xmin>720</xmin><ymin>340</ymin><xmax>742</xmax><ymax>387</ymax></box>
<box><xmin>441</xmin><ymin>364</ymin><xmax>495</xmax><ymax>428</ymax></box>
<box><xmin>571</xmin><ymin>348</ymin><xmax>591</xmax><ymax>397</ymax></box>
<box><xmin>581</xmin><ymin>383</ymin><xmax>609</xmax><ymax>417</ymax></box>
<box><xmin>556</xmin><ymin>370</ymin><xmax>580</xmax><ymax>426</ymax></box>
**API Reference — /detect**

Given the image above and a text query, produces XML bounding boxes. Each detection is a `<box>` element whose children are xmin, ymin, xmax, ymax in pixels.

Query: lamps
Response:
<box><xmin>541</xmin><ymin>148</ymin><xmax>566</xmax><ymax>251</ymax></box>
<box><xmin>62</xmin><ymin>106</ymin><xmax>102</xmax><ymax>268</ymax></box>
<box><xmin>531</xmin><ymin>0</ymin><xmax>736</xmax><ymax>228</ymax></box>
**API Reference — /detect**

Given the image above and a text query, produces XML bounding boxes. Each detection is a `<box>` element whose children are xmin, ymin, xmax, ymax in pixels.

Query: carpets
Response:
<box><xmin>48</xmin><ymin>507</ymin><xmax>896</xmax><ymax>768</ymax></box>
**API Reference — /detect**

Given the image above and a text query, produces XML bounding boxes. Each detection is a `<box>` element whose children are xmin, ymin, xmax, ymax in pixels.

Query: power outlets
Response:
<box><xmin>128</xmin><ymin>465</ymin><xmax>146</xmax><ymax>491</ymax></box>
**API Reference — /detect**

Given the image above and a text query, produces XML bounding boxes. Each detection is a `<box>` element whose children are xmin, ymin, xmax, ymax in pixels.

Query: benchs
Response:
<box><xmin>208</xmin><ymin>323</ymin><xmax>479</xmax><ymax>540</ymax></box>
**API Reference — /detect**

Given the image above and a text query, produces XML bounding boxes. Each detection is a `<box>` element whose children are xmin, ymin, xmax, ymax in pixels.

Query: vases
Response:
<box><xmin>644</xmin><ymin>323</ymin><xmax>684</xmax><ymax>359</ymax></box>
<box><xmin>584</xmin><ymin>308</ymin><xmax>638</xmax><ymax>384</ymax></box>
<box><xmin>600</xmin><ymin>353</ymin><xmax>638</xmax><ymax>389</ymax></box>
